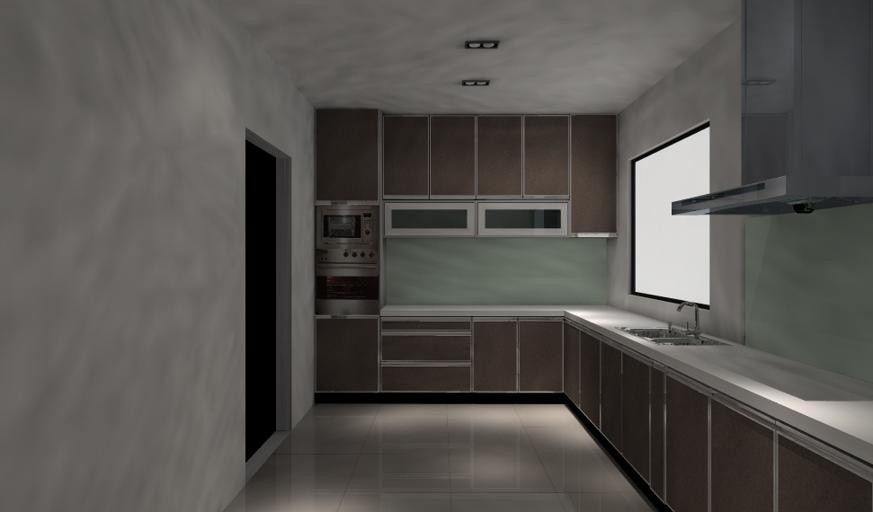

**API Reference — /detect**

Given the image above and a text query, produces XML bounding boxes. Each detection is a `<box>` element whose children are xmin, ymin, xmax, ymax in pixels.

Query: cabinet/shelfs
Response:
<box><xmin>381</xmin><ymin>112</ymin><xmax>476</xmax><ymax>200</ymax></box>
<box><xmin>383</xmin><ymin>319</ymin><xmax>470</xmax><ymax>391</ymax></box>
<box><xmin>565</xmin><ymin>319</ymin><xmax>600</xmax><ymax>426</ymax></box>
<box><xmin>315</xmin><ymin>315</ymin><xmax>379</xmax><ymax>393</ymax></box>
<box><xmin>313</xmin><ymin>105</ymin><xmax>379</xmax><ymax>204</ymax></box>
<box><xmin>572</xmin><ymin>114</ymin><xmax>617</xmax><ymax>236</ymax></box>
<box><xmin>663</xmin><ymin>373</ymin><xmax>776</xmax><ymax>512</ymax></box>
<box><xmin>777</xmin><ymin>436</ymin><xmax>872</xmax><ymax>512</ymax></box>
<box><xmin>477</xmin><ymin>113</ymin><xmax>569</xmax><ymax>200</ymax></box>
<box><xmin>596</xmin><ymin>340</ymin><xmax>651</xmax><ymax>487</ymax></box>
<box><xmin>475</xmin><ymin>322</ymin><xmax>563</xmax><ymax>392</ymax></box>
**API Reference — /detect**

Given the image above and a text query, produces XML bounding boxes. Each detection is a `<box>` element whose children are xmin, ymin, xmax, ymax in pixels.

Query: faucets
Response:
<box><xmin>677</xmin><ymin>302</ymin><xmax>702</xmax><ymax>337</ymax></box>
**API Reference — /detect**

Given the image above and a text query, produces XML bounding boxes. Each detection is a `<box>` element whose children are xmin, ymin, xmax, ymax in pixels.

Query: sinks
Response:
<box><xmin>646</xmin><ymin>337</ymin><xmax>735</xmax><ymax>346</ymax></box>
<box><xmin>614</xmin><ymin>326</ymin><xmax>690</xmax><ymax>338</ymax></box>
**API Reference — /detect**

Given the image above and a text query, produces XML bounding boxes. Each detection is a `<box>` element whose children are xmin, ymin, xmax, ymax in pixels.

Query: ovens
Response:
<box><xmin>314</xmin><ymin>203</ymin><xmax>379</xmax><ymax>317</ymax></box>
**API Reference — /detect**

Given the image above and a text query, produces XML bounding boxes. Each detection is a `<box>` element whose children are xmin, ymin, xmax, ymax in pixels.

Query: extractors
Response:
<box><xmin>673</xmin><ymin>1</ymin><xmax>873</xmax><ymax>214</ymax></box>
